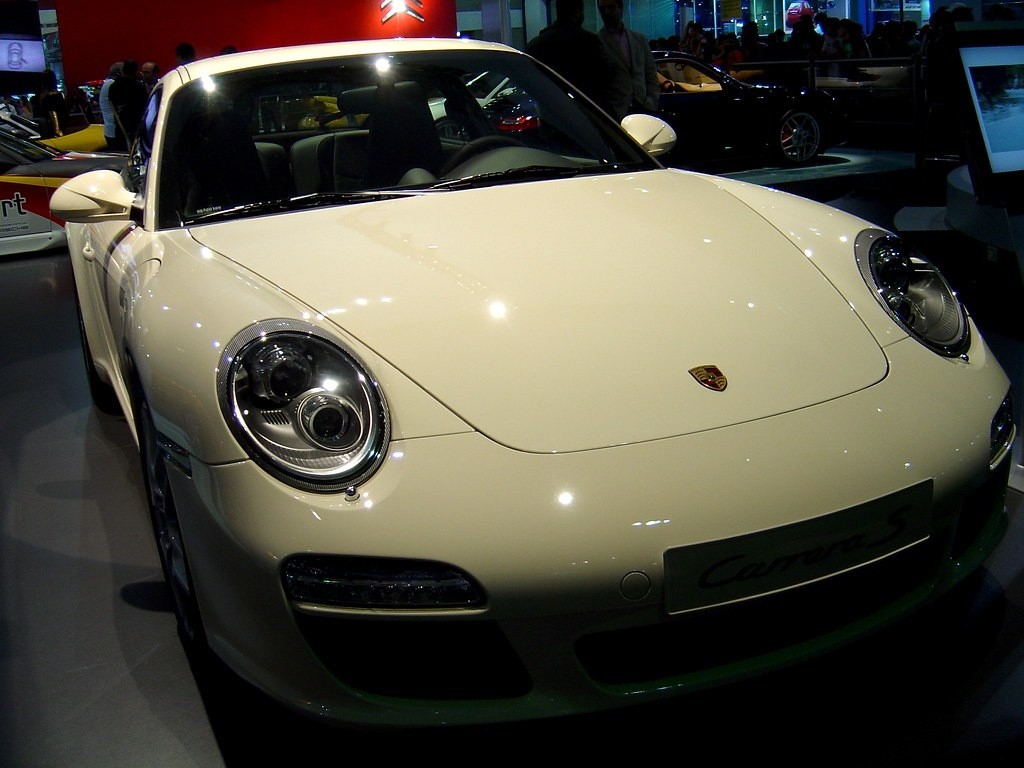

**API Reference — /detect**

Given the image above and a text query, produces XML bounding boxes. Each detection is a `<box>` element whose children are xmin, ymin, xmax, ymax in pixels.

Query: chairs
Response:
<box><xmin>288</xmin><ymin>128</ymin><xmax>371</xmax><ymax>193</ymax></box>
<box><xmin>255</xmin><ymin>141</ymin><xmax>295</xmax><ymax>194</ymax></box>
<box><xmin>330</xmin><ymin>80</ymin><xmax>441</xmax><ymax>190</ymax></box>
<box><xmin>176</xmin><ymin>110</ymin><xmax>271</xmax><ymax>210</ymax></box>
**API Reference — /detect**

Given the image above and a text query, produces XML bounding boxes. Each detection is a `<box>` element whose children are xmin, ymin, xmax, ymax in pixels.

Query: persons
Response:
<box><xmin>222</xmin><ymin>46</ymin><xmax>282</xmax><ymax>133</ymax></box>
<box><xmin>99</xmin><ymin>62</ymin><xmax>124</xmax><ymax>152</ymax></box>
<box><xmin>141</xmin><ymin>62</ymin><xmax>161</xmax><ymax>91</ymax></box>
<box><xmin>30</xmin><ymin>90</ymin><xmax>43</xmax><ymax>118</ymax></box>
<box><xmin>866</xmin><ymin>5</ymin><xmax>973</xmax><ymax>57</ymax></box>
<box><xmin>528</xmin><ymin>0</ymin><xmax>661</xmax><ymax>125</ymax></box>
<box><xmin>108</xmin><ymin>60</ymin><xmax>147</xmax><ymax>153</ymax></box>
<box><xmin>175</xmin><ymin>44</ymin><xmax>196</xmax><ymax>65</ymax></box>
<box><xmin>649</xmin><ymin>14</ymin><xmax>871</xmax><ymax>82</ymax></box>
<box><xmin>0</xmin><ymin>96</ymin><xmax>33</xmax><ymax>118</ymax></box>
<box><xmin>41</xmin><ymin>70</ymin><xmax>68</xmax><ymax>137</ymax></box>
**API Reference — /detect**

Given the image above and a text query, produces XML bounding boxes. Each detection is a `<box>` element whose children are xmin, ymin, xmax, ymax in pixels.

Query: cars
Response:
<box><xmin>651</xmin><ymin>49</ymin><xmax>836</xmax><ymax>171</ymax></box>
<box><xmin>0</xmin><ymin>79</ymin><xmax>129</xmax><ymax>257</ymax></box>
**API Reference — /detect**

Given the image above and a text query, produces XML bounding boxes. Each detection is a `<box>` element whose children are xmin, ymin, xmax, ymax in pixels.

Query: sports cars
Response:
<box><xmin>47</xmin><ymin>30</ymin><xmax>1019</xmax><ymax>729</ymax></box>
